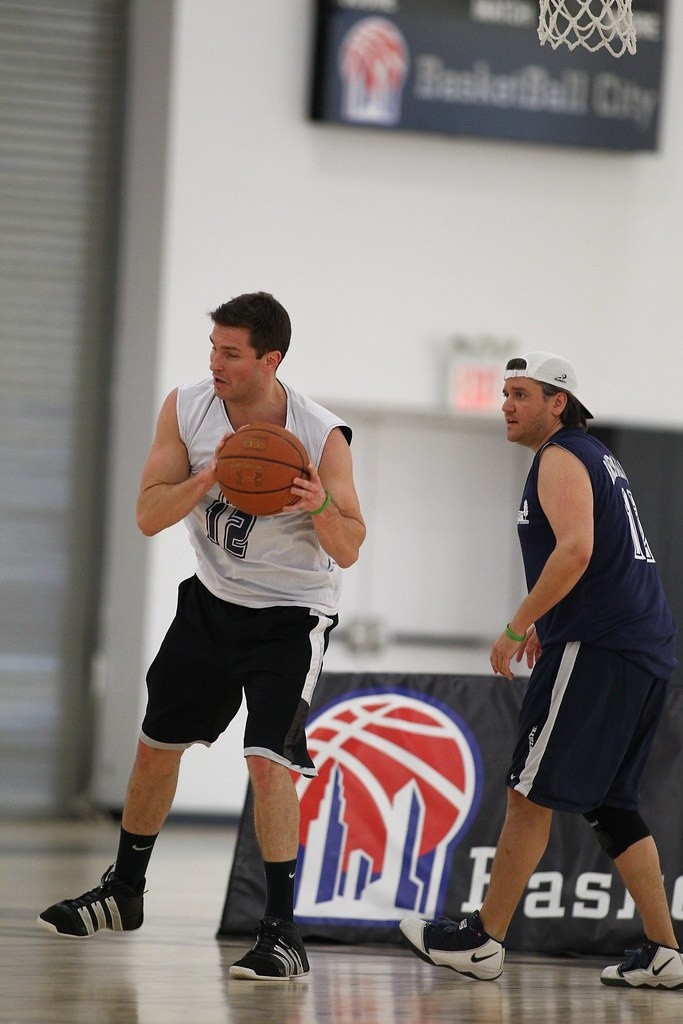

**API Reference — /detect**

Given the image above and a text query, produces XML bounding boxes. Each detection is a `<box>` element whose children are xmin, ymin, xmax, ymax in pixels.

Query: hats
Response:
<box><xmin>504</xmin><ymin>350</ymin><xmax>594</xmax><ymax>419</ymax></box>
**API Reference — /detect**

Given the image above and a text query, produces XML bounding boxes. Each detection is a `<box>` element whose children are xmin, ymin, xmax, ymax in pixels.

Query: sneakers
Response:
<box><xmin>229</xmin><ymin>921</ymin><xmax>310</xmax><ymax>980</ymax></box>
<box><xmin>398</xmin><ymin>909</ymin><xmax>505</xmax><ymax>981</ymax></box>
<box><xmin>600</xmin><ymin>934</ymin><xmax>683</xmax><ymax>990</ymax></box>
<box><xmin>38</xmin><ymin>862</ymin><xmax>144</xmax><ymax>938</ymax></box>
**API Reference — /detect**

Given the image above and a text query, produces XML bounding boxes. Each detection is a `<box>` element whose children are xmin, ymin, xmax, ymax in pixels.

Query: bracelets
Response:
<box><xmin>308</xmin><ymin>489</ymin><xmax>331</xmax><ymax>514</ymax></box>
<box><xmin>506</xmin><ymin>623</ymin><xmax>527</xmax><ymax>641</ymax></box>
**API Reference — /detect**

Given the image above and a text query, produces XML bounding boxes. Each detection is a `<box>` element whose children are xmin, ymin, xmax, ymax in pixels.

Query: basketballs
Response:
<box><xmin>214</xmin><ymin>420</ymin><xmax>312</xmax><ymax>516</ymax></box>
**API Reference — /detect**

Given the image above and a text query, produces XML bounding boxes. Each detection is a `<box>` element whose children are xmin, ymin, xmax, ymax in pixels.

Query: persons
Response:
<box><xmin>397</xmin><ymin>352</ymin><xmax>683</xmax><ymax>989</ymax></box>
<box><xmin>37</xmin><ymin>293</ymin><xmax>366</xmax><ymax>980</ymax></box>
